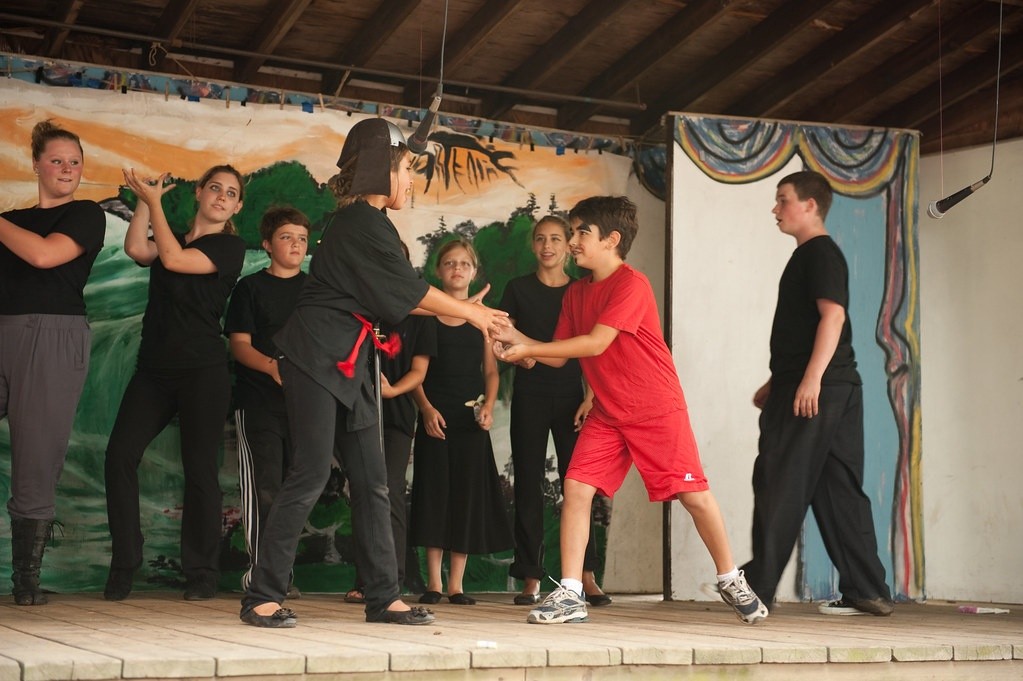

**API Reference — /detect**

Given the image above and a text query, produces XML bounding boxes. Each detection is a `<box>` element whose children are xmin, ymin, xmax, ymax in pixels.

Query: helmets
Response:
<box><xmin>337</xmin><ymin>117</ymin><xmax>410</xmax><ymax>171</ymax></box>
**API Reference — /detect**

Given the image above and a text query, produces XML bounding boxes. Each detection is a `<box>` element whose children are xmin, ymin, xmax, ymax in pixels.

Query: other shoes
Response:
<box><xmin>240</xmin><ymin>607</ymin><xmax>298</xmax><ymax>629</ymax></box>
<box><xmin>419</xmin><ymin>590</ymin><xmax>443</xmax><ymax>605</ymax></box>
<box><xmin>585</xmin><ymin>592</ymin><xmax>612</xmax><ymax>606</ymax></box>
<box><xmin>284</xmin><ymin>585</ymin><xmax>301</xmax><ymax>600</ymax></box>
<box><xmin>448</xmin><ymin>592</ymin><xmax>477</xmax><ymax>605</ymax></box>
<box><xmin>514</xmin><ymin>592</ymin><xmax>542</xmax><ymax>606</ymax></box>
<box><xmin>103</xmin><ymin>556</ymin><xmax>145</xmax><ymax>602</ymax></box>
<box><xmin>345</xmin><ymin>589</ymin><xmax>367</xmax><ymax>604</ymax></box>
<box><xmin>366</xmin><ymin>606</ymin><xmax>435</xmax><ymax>625</ymax></box>
<box><xmin>184</xmin><ymin>581</ymin><xmax>216</xmax><ymax>602</ymax></box>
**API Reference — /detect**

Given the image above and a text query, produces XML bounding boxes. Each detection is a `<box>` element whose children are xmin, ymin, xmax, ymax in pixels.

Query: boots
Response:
<box><xmin>7</xmin><ymin>511</ymin><xmax>64</xmax><ymax>607</ymax></box>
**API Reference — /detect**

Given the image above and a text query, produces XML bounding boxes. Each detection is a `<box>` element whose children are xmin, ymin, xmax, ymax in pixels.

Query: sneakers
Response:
<box><xmin>817</xmin><ymin>597</ymin><xmax>891</xmax><ymax>616</ymax></box>
<box><xmin>699</xmin><ymin>582</ymin><xmax>726</xmax><ymax>603</ymax></box>
<box><xmin>719</xmin><ymin>569</ymin><xmax>769</xmax><ymax>626</ymax></box>
<box><xmin>526</xmin><ymin>576</ymin><xmax>589</xmax><ymax>624</ymax></box>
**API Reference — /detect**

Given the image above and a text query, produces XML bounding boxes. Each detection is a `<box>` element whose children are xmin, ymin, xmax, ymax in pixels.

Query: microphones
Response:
<box><xmin>407</xmin><ymin>95</ymin><xmax>443</xmax><ymax>154</ymax></box>
<box><xmin>926</xmin><ymin>174</ymin><xmax>991</xmax><ymax>219</ymax></box>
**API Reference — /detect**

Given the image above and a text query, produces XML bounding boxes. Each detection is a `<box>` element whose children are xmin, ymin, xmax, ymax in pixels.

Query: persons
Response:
<box><xmin>223</xmin><ymin>201</ymin><xmax>310</xmax><ymax>599</ymax></box>
<box><xmin>0</xmin><ymin>118</ymin><xmax>107</xmax><ymax>604</ymax></box>
<box><xmin>344</xmin><ymin>240</ymin><xmax>500</xmax><ymax>605</ymax></box>
<box><xmin>501</xmin><ymin>215</ymin><xmax>613</xmax><ymax>606</ymax></box>
<box><xmin>698</xmin><ymin>170</ymin><xmax>892</xmax><ymax>615</ymax></box>
<box><xmin>489</xmin><ymin>196</ymin><xmax>769</xmax><ymax>627</ymax></box>
<box><xmin>239</xmin><ymin>118</ymin><xmax>512</xmax><ymax>627</ymax></box>
<box><xmin>103</xmin><ymin>166</ymin><xmax>247</xmax><ymax>601</ymax></box>
